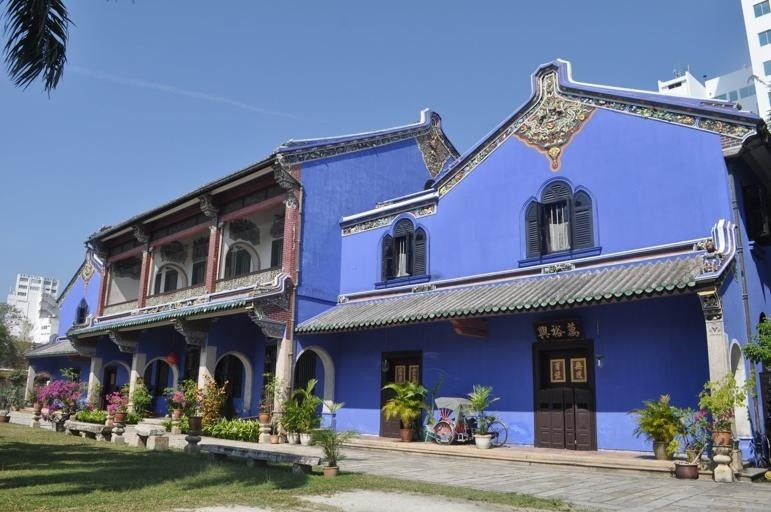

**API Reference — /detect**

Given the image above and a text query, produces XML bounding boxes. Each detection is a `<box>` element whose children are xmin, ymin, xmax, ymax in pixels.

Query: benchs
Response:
<box><xmin>63</xmin><ymin>418</ymin><xmax>112</xmax><ymax>442</ymax></box>
<box><xmin>128</xmin><ymin>424</ymin><xmax>169</xmax><ymax>452</ymax></box>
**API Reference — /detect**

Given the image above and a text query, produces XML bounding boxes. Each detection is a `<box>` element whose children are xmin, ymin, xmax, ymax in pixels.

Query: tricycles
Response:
<box><xmin>424</xmin><ymin>397</ymin><xmax>507</xmax><ymax>448</ymax></box>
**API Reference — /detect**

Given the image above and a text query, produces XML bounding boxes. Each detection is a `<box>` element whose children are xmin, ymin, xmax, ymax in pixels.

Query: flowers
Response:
<box><xmin>106</xmin><ymin>392</ymin><xmax>129</xmax><ymax>416</ymax></box>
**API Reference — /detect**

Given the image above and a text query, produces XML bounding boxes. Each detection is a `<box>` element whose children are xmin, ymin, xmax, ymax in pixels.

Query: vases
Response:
<box><xmin>114</xmin><ymin>413</ymin><xmax>127</xmax><ymax>423</ymax></box>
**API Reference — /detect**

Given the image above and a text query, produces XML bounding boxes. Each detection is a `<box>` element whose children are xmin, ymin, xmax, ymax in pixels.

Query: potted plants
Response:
<box><xmin>26</xmin><ymin>378</ymin><xmax>44</xmax><ymax>409</ymax></box>
<box><xmin>466</xmin><ymin>384</ymin><xmax>500</xmax><ymax>450</ymax></box>
<box><xmin>0</xmin><ymin>384</ymin><xmax>23</xmax><ymax>423</ymax></box>
<box><xmin>628</xmin><ymin>368</ymin><xmax>759</xmax><ymax>479</ymax></box>
<box><xmin>379</xmin><ymin>383</ymin><xmax>428</xmax><ymax>441</ymax></box>
<box><xmin>258</xmin><ymin>373</ymin><xmax>359</xmax><ymax>477</ymax></box>
<box><xmin>164</xmin><ymin>381</ymin><xmax>194</xmax><ymax>421</ymax></box>
